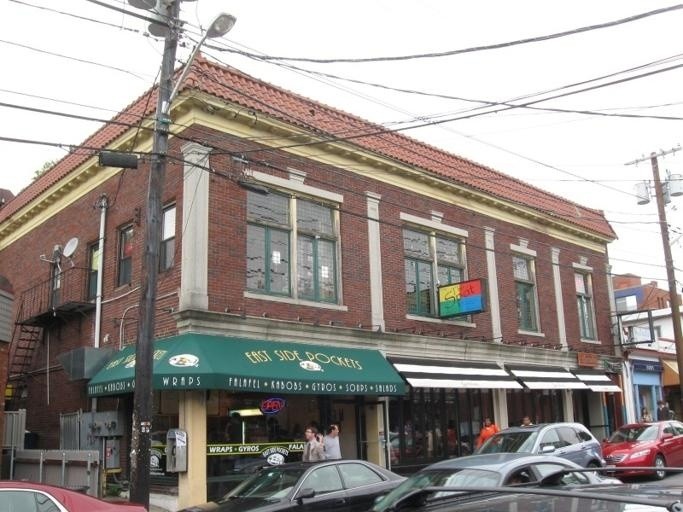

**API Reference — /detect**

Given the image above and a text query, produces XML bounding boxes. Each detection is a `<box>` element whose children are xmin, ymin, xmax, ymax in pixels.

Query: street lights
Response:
<box><xmin>129</xmin><ymin>0</ymin><xmax>236</xmax><ymax>511</ymax></box>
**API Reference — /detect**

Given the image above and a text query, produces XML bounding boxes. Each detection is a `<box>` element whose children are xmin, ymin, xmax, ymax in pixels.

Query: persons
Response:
<box><xmin>300</xmin><ymin>424</ymin><xmax>327</xmax><ymax>463</ymax></box>
<box><xmin>448</xmin><ymin>419</ymin><xmax>457</xmax><ymax>452</ymax></box>
<box><xmin>640</xmin><ymin>407</ymin><xmax>654</xmax><ymax>422</ymax></box>
<box><xmin>477</xmin><ymin>417</ymin><xmax>502</xmax><ymax>451</ymax></box>
<box><xmin>224</xmin><ymin>412</ymin><xmax>243</xmax><ymax>443</ymax></box>
<box><xmin>657</xmin><ymin>399</ymin><xmax>675</xmax><ymax>421</ymax></box>
<box><xmin>521</xmin><ymin>414</ymin><xmax>533</xmax><ymax>427</ymax></box>
<box><xmin>321</xmin><ymin>425</ymin><xmax>341</xmax><ymax>460</ymax></box>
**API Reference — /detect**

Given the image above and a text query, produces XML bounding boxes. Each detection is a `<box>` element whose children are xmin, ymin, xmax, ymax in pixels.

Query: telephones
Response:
<box><xmin>172</xmin><ymin>445</ymin><xmax>176</xmax><ymax>456</ymax></box>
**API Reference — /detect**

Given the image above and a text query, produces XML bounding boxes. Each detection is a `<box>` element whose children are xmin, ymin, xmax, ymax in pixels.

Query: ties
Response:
<box><xmin>306</xmin><ymin>443</ymin><xmax>310</xmax><ymax>460</ymax></box>
